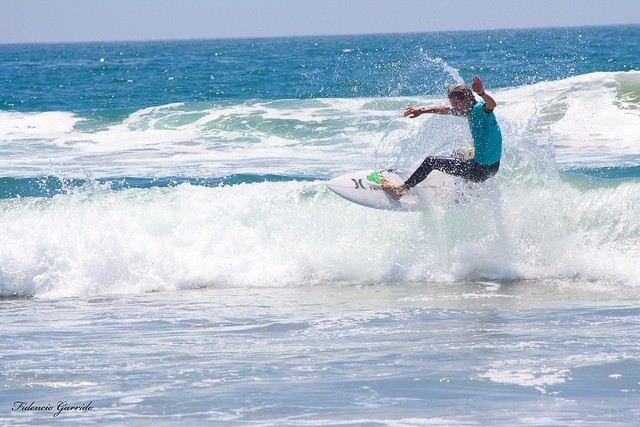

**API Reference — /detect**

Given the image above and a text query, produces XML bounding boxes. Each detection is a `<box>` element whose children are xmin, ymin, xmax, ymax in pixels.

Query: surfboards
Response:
<box><xmin>325</xmin><ymin>169</ymin><xmax>479</xmax><ymax>212</ymax></box>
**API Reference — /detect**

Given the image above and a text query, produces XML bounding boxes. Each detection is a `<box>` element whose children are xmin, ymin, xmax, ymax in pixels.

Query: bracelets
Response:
<box><xmin>477</xmin><ymin>90</ymin><xmax>485</xmax><ymax>96</ymax></box>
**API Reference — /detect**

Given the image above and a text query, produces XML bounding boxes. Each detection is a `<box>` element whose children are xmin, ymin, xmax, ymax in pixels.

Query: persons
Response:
<box><xmin>381</xmin><ymin>75</ymin><xmax>503</xmax><ymax>201</ymax></box>
